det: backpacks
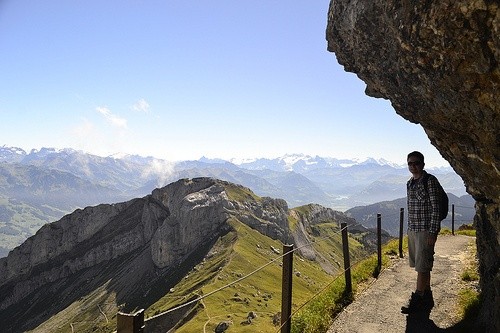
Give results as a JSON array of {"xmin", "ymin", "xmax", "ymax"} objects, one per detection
[{"xmin": 424, "ymin": 174, "xmax": 448, "ymax": 221}]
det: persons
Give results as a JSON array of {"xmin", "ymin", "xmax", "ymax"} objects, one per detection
[{"xmin": 401, "ymin": 151, "xmax": 441, "ymax": 312}]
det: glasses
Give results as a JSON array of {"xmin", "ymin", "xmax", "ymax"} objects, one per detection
[{"xmin": 408, "ymin": 162, "xmax": 420, "ymax": 166}]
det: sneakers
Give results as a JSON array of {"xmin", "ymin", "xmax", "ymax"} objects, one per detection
[{"xmin": 401, "ymin": 291, "xmax": 434, "ymax": 312}]
